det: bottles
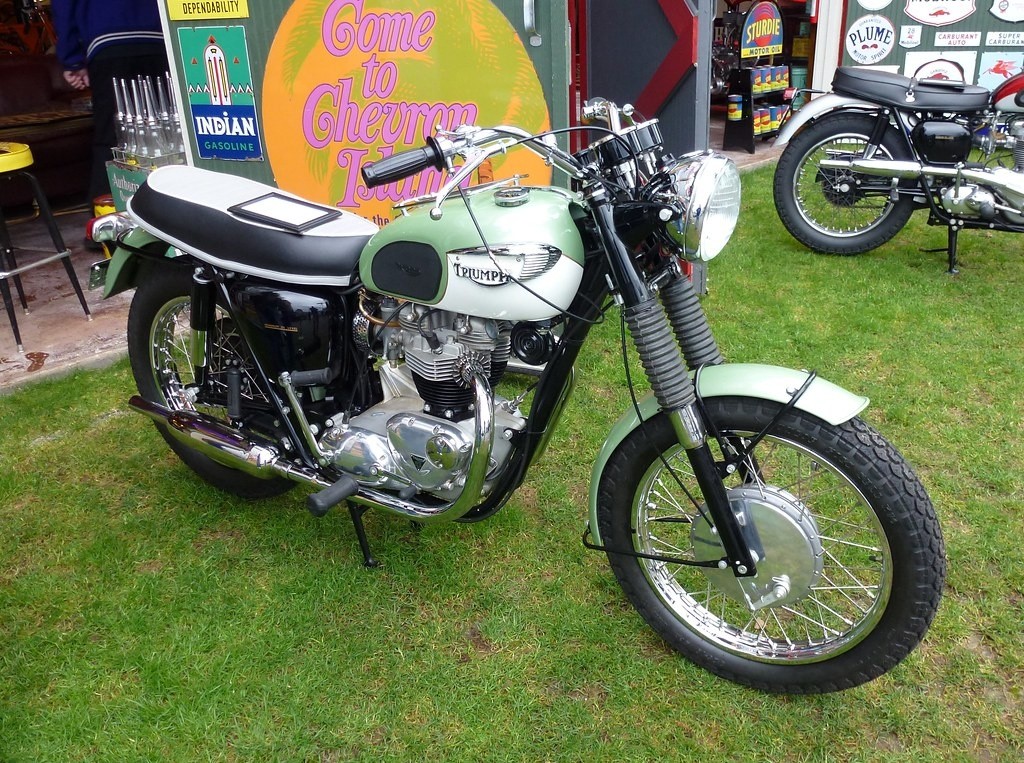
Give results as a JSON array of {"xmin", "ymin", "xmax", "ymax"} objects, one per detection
[{"xmin": 112, "ymin": 72, "xmax": 188, "ymax": 171}]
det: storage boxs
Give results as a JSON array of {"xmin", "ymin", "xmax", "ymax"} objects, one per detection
[
  {"xmin": 104, "ymin": 160, "xmax": 148, "ymax": 213},
  {"xmin": 793, "ymin": 35, "xmax": 811, "ymax": 56}
]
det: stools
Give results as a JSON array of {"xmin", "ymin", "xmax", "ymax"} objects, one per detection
[{"xmin": 0, "ymin": 141, "xmax": 94, "ymax": 353}]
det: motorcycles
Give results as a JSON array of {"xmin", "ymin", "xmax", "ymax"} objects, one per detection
[
  {"xmin": 770, "ymin": 65, "xmax": 1024, "ymax": 274},
  {"xmin": 84, "ymin": 95, "xmax": 947, "ymax": 695}
]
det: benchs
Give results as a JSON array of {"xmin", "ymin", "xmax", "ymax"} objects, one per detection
[{"xmin": 0, "ymin": 53, "xmax": 98, "ymax": 210}]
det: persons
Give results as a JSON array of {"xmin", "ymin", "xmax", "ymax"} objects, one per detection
[{"xmin": 49, "ymin": 0, "xmax": 174, "ymax": 252}]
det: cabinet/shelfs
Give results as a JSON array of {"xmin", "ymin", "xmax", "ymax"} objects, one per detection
[{"xmin": 724, "ymin": 69, "xmax": 793, "ymax": 155}]
